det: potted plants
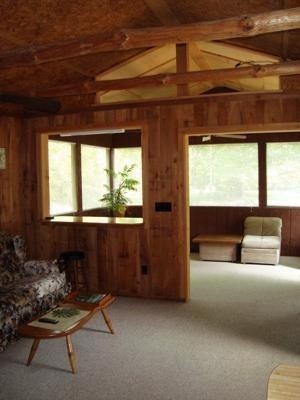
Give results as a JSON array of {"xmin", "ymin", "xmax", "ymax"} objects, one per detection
[{"xmin": 98, "ymin": 164, "xmax": 140, "ymax": 217}]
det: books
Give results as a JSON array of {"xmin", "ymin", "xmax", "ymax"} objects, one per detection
[{"xmin": 76, "ymin": 292, "xmax": 101, "ymax": 304}]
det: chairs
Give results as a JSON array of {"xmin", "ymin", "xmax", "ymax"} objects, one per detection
[{"xmin": 240, "ymin": 215, "xmax": 283, "ymax": 265}]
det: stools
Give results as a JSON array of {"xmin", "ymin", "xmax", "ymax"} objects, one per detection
[{"xmin": 60, "ymin": 251, "xmax": 89, "ymax": 292}]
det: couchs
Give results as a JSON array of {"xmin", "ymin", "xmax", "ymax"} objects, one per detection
[{"xmin": 0, "ymin": 231, "xmax": 72, "ymax": 355}]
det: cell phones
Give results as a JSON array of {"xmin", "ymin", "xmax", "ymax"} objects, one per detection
[{"xmin": 38, "ymin": 318, "xmax": 58, "ymax": 324}]
